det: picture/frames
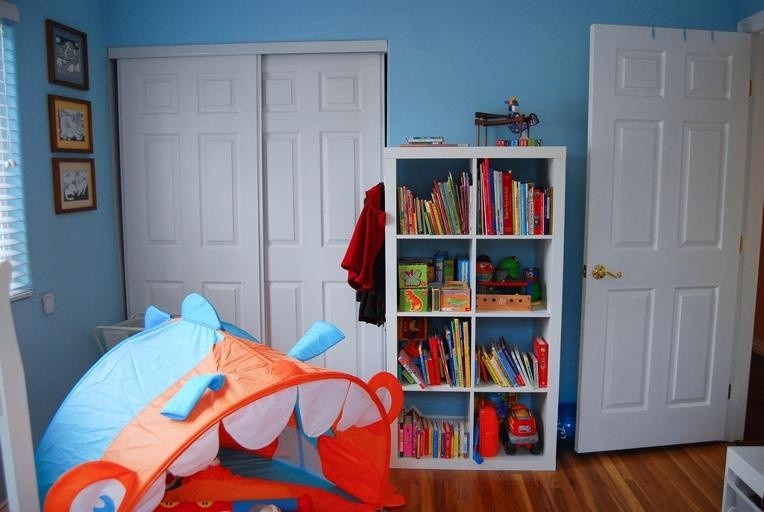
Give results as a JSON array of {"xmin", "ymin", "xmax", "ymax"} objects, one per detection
[{"xmin": 48, "ymin": 20, "xmax": 98, "ymax": 216}]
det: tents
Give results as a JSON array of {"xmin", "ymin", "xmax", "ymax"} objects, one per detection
[{"xmin": 29, "ymin": 289, "xmax": 412, "ymax": 512}]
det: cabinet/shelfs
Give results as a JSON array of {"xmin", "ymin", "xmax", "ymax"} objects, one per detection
[
  {"xmin": 385, "ymin": 146, "xmax": 560, "ymax": 474},
  {"xmin": 112, "ymin": 49, "xmax": 385, "ymax": 405}
]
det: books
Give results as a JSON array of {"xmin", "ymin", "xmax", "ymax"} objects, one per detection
[
  {"xmin": 397, "ymin": 402, "xmax": 468, "ymax": 461},
  {"xmin": 395, "ymin": 136, "xmax": 551, "ymax": 391}
]
